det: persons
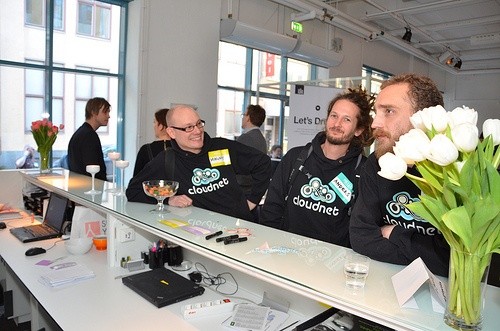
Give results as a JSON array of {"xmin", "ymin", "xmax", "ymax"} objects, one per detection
[
  {"xmin": 125, "ymin": 101, "xmax": 272, "ymax": 224},
  {"xmin": 348, "ymin": 73, "xmax": 500, "ymax": 287},
  {"xmin": 261, "ymin": 84, "xmax": 376, "ymax": 249},
  {"xmin": 132, "ymin": 108, "xmax": 172, "ymax": 178},
  {"xmin": 268, "ymin": 144, "xmax": 282, "ymax": 158},
  {"xmin": 67, "ymin": 97, "xmax": 112, "ymax": 182},
  {"xmin": 236, "ymin": 105, "xmax": 268, "ymax": 156}
]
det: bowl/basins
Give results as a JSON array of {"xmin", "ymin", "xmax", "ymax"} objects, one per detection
[
  {"xmin": 92, "ymin": 235, "xmax": 107, "ymax": 250},
  {"xmin": 66, "ymin": 238, "xmax": 93, "ymax": 255}
]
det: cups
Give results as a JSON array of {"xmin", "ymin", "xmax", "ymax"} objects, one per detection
[{"xmin": 342, "ymin": 252, "xmax": 370, "ymax": 289}]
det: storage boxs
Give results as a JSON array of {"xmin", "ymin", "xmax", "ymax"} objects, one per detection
[{"xmin": 42, "ymin": 198, "xmax": 49, "ymax": 220}]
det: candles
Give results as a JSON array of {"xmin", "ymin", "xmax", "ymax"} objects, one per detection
[
  {"xmin": 108, "ymin": 151, "xmax": 121, "ymax": 160},
  {"xmin": 86, "ymin": 165, "xmax": 100, "ymax": 174},
  {"xmin": 116, "ymin": 159, "xmax": 129, "ymax": 169}
]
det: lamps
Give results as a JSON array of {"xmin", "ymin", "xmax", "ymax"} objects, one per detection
[
  {"xmin": 453, "ymin": 57, "xmax": 463, "ymax": 70},
  {"xmin": 402, "ymin": 26, "xmax": 413, "ymax": 43}
]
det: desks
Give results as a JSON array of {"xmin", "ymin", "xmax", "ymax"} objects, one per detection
[{"xmin": 0, "ymin": 216, "xmax": 312, "ymax": 331}]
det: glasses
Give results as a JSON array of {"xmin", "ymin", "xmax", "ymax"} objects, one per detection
[
  {"xmin": 169, "ymin": 119, "xmax": 206, "ymax": 133},
  {"xmin": 241, "ymin": 114, "xmax": 247, "ymax": 117}
]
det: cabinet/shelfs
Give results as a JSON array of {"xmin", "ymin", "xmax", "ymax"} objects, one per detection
[{"xmin": 23, "ymin": 191, "xmax": 50, "ymax": 217}]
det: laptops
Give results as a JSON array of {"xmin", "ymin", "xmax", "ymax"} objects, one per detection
[
  {"xmin": 121, "ymin": 267, "xmax": 205, "ymax": 308},
  {"xmin": 9, "ymin": 191, "xmax": 69, "ymax": 243}
]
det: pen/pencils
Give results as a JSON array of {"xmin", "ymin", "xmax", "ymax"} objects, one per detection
[{"xmin": 149, "ymin": 240, "xmax": 167, "ymax": 253}]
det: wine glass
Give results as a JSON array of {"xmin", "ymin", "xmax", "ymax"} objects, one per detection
[
  {"xmin": 85, "ymin": 165, "xmax": 102, "ymax": 194},
  {"xmin": 115, "ymin": 160, "xmax": 129, "ymax": 195},
  {"xmin": 142, "ymin": 179, "xmax": 179, "ymax": 214},
  {"xmin": 107, "ymin": 153, "xmax": 121, "ymax": 192}
]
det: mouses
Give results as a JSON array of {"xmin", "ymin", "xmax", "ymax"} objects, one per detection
[
  {"xmin": 25, "ymin": 247, "xmax": 46, "ymax": 257},
  {"xmin": 187, "ymin": 270, "xmax": 202, "ymax": 283}
]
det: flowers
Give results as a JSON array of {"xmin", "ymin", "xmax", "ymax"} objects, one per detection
[
  {"xmin": 374, "ymin": 102, "xmax": 500, "ymax": 325},
  {"xmin": 30, "ymin": 117, "xmax": 65, "ymax": 170}
]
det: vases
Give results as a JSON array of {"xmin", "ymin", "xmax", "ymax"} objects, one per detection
[
  {"xmin": 442, "ymin": 246, "xmax": 492, "ymax": 331},
  {"xmin": 40, "ymin": 149, "xmax": 53, "ymax": 174}
]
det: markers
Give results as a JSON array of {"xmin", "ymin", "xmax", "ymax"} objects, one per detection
[
  {"xmin": 224, "ymin": 237, "xmax": 247, "ymax": 245},
  {"xmin": 215, "ymin": 235, "xmax": 239, "ymax": 242},
  {"xmin": 206, "ymin": 231, "xmax": 223, "ymax": 240}
]
